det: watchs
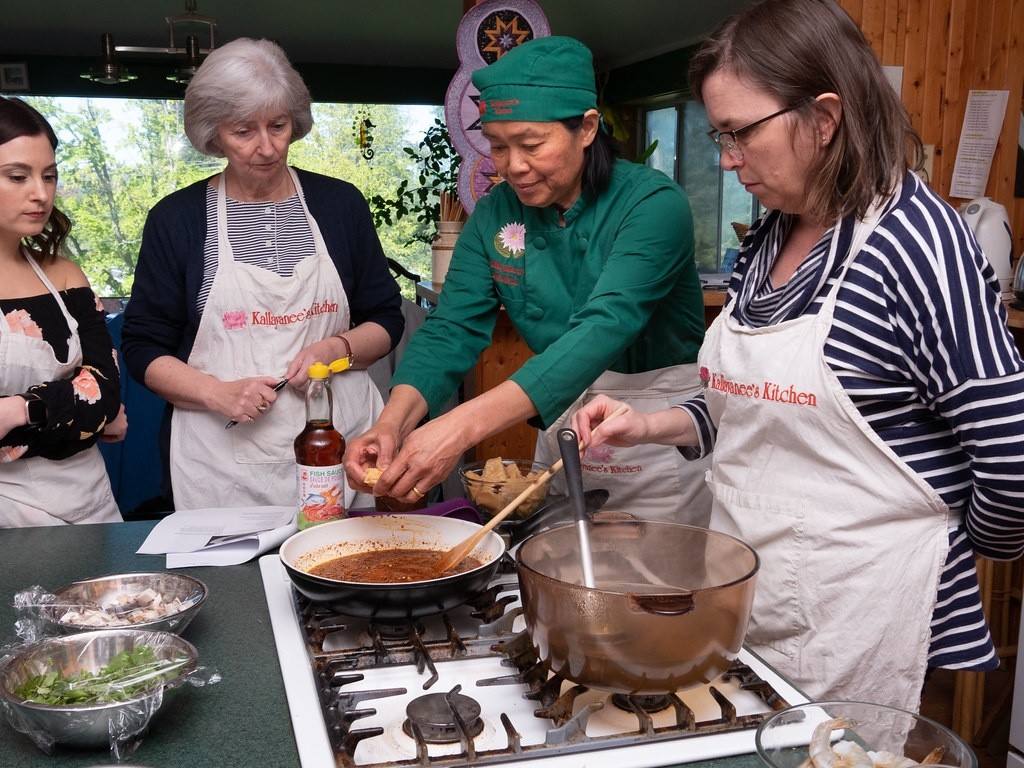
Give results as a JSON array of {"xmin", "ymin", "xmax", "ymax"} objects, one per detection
[
  {"xmin": 336, "ymin": 336, "xmax": 354, "ymax": 368},
  {"xmin": 15, "ymin": 393, "xmax": 47, "ymax": 424}
]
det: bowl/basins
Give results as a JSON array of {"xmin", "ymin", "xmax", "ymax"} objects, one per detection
[
  {"xmin": 42, "ymin": 571, "xmax": 208, "ymax": 637},
  {"xmin": 755, "ymin": 702, "xmax": 978, "ymax": 768},
  {"xmin": 0, "ymin": 629, "xmax": 198, "ymax": 744},
  {"xmin": 459, "ymin": 459, "xmax": 554, "ymax": 523}
]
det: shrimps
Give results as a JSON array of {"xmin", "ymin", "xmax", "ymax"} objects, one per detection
[
  {"xmin": 868, "ymin": 743, "xmax": 960, "ymax": 768},
  {"xmin": 809, "ymin": 715, "xmax": 875, "ymax": 768}
]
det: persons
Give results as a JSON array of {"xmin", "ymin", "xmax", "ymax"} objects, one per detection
[
  {"xmin": 570, "ymin": 0, "xmax": 1024, "ymax": 757},
  {"xmin": 0, "ymin": 96, "xmax": 128, "ymax": 529},
  {"xmin": 345, "ymin": 36, "xmax": 711, "ymax": 528},
  {"xmin": 120, "ymin": 38, "xmax": 406, "ymax": 513}
]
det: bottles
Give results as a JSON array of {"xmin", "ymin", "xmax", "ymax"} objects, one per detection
[
  {"xmin": 432, "ymin": 221, "xmax": 464, "ymax": 286},
  {"xmin": 294, "ymin": 357, "xmax": 352, "ymax": 532}
]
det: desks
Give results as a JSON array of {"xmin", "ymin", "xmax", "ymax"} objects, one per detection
[
  {"xmin": 417, "ymin": 281, "xmax": 725, "ymax": 476},
  {"xmin": 0, "ymin": 511, "xmax": 884, "ymax": 768}
]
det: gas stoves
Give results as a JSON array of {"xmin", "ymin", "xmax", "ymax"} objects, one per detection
[{"xmin": 257, "ymin": 528, "xmax": 841, "ymax": 768}]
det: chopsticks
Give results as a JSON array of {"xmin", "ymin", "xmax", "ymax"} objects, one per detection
[{"xmin": 439, "ymin": 190, "xmax": 463, "ymax": 221}]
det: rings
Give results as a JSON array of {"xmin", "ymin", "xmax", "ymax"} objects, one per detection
[
  {"xmin": 257, "ymin": 400, "xmax": 267, "ymax": 411},
  {"xmin": 247, "ymin": 417, "xmax": 250, "ymax": 424},
  {"xmin": 412, "ymin": 486, "xmax": 425, "ymax": 497}
]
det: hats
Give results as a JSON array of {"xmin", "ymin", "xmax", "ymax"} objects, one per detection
[{"xmin": 472, "ymin": 35, "xmax": 598, "ymax": 123}]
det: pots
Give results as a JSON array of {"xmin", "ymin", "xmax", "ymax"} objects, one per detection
[
  {"xmin": 515, "ymin": 511, "xmax": 760, "ymax": 695},
  {"xmin": 280, "ymin": 489, "xmax": 609, "ymax": 621}
]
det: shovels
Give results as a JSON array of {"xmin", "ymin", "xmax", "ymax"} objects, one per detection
[{"xmin": 434, "ymin": 403, "xmax": 630, "ymax": 572}]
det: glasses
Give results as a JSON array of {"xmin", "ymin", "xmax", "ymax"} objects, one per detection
[{"xmin": 706, "ymin": 100, "xmax": 810, "ymax": 161}]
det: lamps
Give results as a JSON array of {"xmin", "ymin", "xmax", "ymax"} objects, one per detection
[{"xmin": 80, "ymin": 1, "xmax": 225, "ymax": 86}]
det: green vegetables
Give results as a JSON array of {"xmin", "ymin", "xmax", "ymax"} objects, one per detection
[{"xmin": 13, "ymin": 642, "xmax": 180, "ymax": 708}]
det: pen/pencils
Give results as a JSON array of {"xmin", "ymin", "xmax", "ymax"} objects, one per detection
[
  {"xmin": 703, "ymin": 285, "xmax": 728, "ymax": 290},
  {"xmin": 225, "ymin": 379, "xmax": 288, "ymax": 431}
]
